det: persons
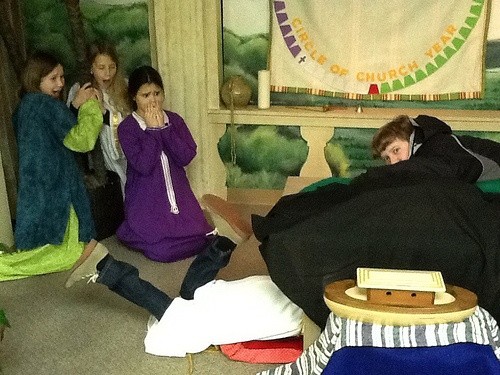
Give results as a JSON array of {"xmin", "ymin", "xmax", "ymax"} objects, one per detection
[
  {"xmin": 66, "ymin": 39, "xmax": 499, "ymax": 357},
  {"xmin": 0, "ymin": 56, "xmax": 104, "ymax": 282}
]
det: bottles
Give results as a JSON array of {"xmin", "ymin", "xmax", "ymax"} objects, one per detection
[{"xmin": 222, "ymin": 75, "xmax": 252, "ymax": 108}]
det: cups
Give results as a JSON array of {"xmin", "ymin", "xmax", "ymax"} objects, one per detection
[{"xmin": 258, "ymin": 69, "xmax": 271, "ymax": 109}]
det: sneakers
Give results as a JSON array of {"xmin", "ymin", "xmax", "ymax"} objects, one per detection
[
  {"xmin": 63, "ymin": 238, "xmax": 109, "ymax": 289},
  {"xmin": 201, "ymin": 193, "xmax": 254, "ymax": 248}
]
